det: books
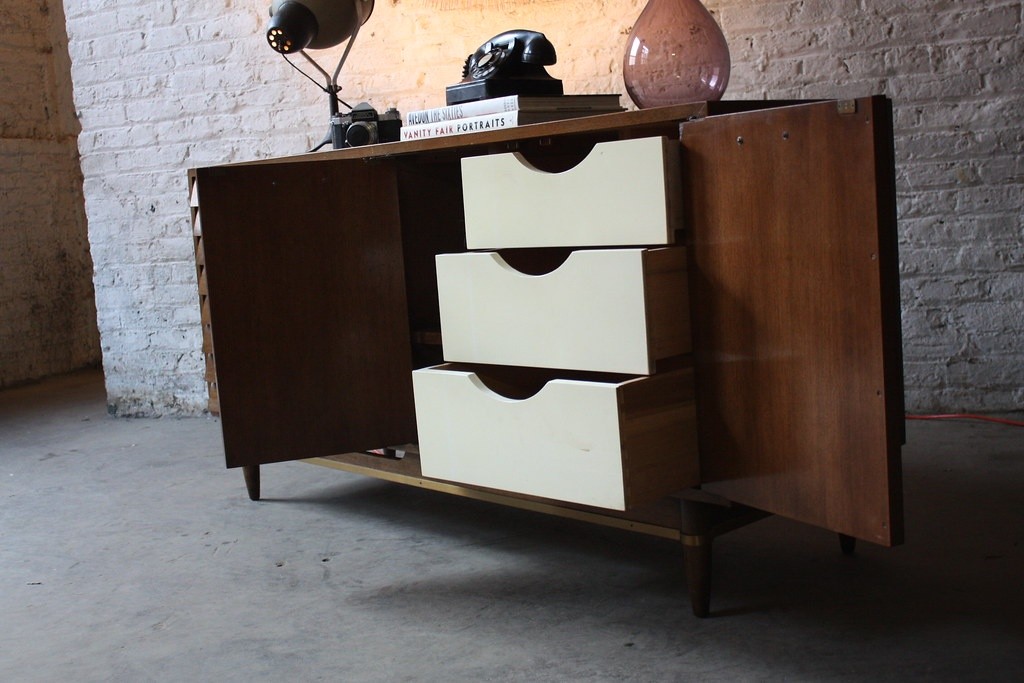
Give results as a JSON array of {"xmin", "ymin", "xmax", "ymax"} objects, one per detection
[{"xmin": 400, "ymin": 94, "xmax": 628, "ymax": 142}]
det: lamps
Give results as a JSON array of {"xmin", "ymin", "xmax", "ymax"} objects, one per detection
[{"xmin": 265, "ymin": 0, "xmax": 375, "ymax": 152}]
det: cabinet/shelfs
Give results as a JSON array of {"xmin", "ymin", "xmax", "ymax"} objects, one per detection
[{"xmin": 185, "ymin": 95, "xmax": 909, "ymax": 620}]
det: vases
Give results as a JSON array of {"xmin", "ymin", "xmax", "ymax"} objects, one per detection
[{"xmin": 622, "ymin": 0, "xmax": 731, "ymax": 109}]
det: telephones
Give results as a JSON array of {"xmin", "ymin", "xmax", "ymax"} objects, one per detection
[{"xmin": 446, "ymin": 30, "xmax": 564, "ymax": 105}]
